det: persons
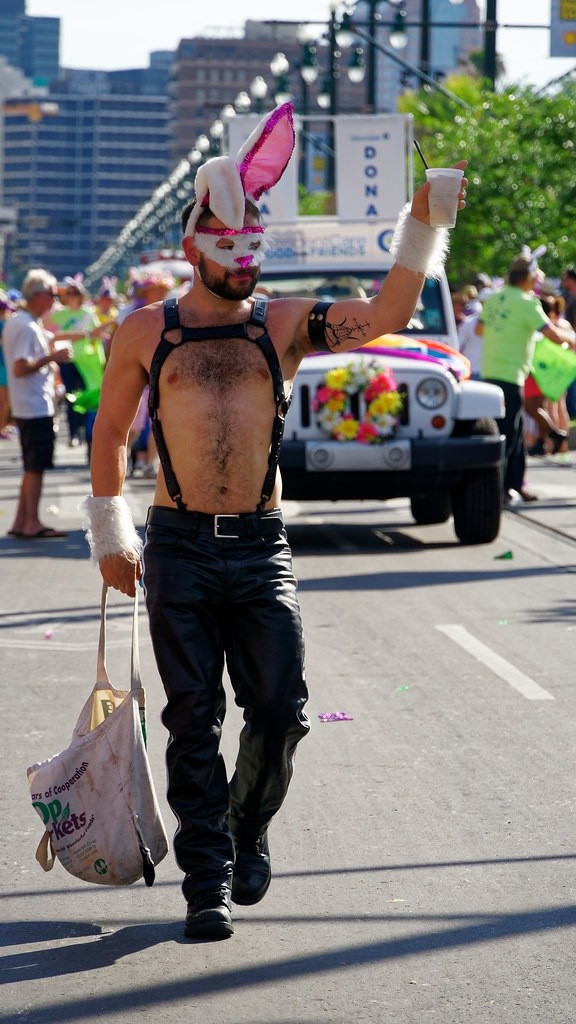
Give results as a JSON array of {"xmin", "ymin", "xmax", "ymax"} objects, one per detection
[
  {"xmin": 85, "ymin": 159, "xmax": 470, "ymax": 939},
  {"xmin": 0, "ymin": 253, "xmax": 576, "ymax": 539}
]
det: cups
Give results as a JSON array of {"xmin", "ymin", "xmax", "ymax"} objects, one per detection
[
  {"xmin": 426, "ymin": 167, "xmax": 464, "ymax": 228},
  {"xmin": 54, "ymin": 339, "xmax": 70, "ymax": 351}
]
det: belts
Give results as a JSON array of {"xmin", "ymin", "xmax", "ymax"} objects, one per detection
[{"xmin": 146, "ymin": 506, "xmax": 283, "ymax": 538}]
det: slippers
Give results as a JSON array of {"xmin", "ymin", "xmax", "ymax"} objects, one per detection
[{"xmin": 7, "ymin": 527, "xmax": 67, "ymax": 538}]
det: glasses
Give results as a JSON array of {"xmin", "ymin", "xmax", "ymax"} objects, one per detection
[{"xmin": 50, "ymin": 291, "xmax": 61, "ymax": 300}]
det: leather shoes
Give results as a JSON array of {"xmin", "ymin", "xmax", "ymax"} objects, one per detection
[
  {"xmin": 183, "ymin": 874, "xmax": 234, "ymax": 937},
  {"xmin": 227, "ymin": 824, "xmax": 271, "ymax": 904}
]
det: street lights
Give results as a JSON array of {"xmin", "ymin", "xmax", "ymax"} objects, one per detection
[{"xmin": 84, "ymin": 0, "xmax": 409, "ymax": 281}]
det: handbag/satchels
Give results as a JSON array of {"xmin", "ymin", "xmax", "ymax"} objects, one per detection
[{"xmin": 26, "ymin": 581, "xmax": 170, "ymax": 888}]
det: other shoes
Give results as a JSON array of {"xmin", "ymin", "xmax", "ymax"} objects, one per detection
[
  {"xmin": 505, "ymin": 486, "xmax": 539, "ymax": 503},
  {"xmin": 130, "ymin": 461, "xmax": 157, "ymax": 480}
]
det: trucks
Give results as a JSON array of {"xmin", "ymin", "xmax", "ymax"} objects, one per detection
[{"xmin": 220, "ymin": 112, "xmax": 509, "ymax": 545}]
